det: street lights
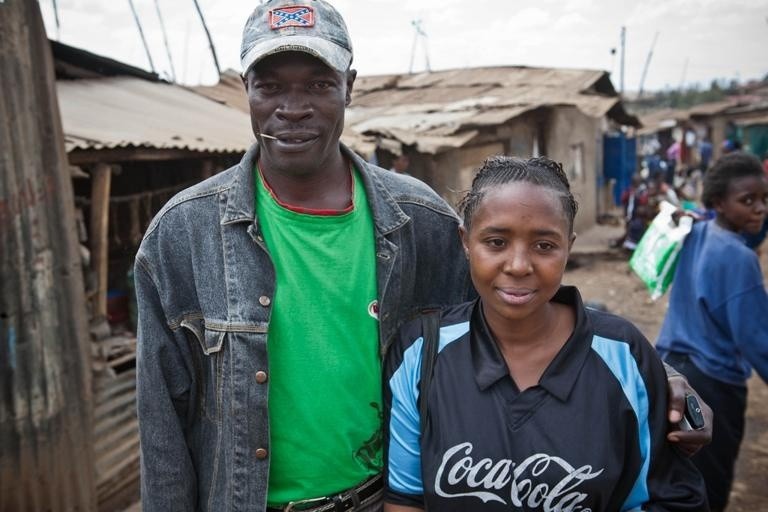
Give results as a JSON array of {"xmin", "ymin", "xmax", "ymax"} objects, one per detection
[{"xmin": 629, "ymin": 203, "xmax": 693, "ymax": 301}]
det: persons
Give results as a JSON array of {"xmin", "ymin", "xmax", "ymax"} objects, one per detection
[
  {"xmin": 131, "ymin": 0, "xmax": 718, "ymax": 512},
  {"xmin": 376, "ymin": 151, "xmax": 719, "ymax": 512},
  {"xmin": 649, "ymin": 153, "xmax": 768, "ymax": 510}
]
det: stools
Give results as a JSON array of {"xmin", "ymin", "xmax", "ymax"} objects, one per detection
[{"xmin": 267, "ymin": 472, "xmax": 383, "ymax": 512}]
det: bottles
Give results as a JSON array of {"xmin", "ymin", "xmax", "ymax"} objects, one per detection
[{"xmin": 240, "ymin": 0, "xmax": 352, "ymax": 78}]
